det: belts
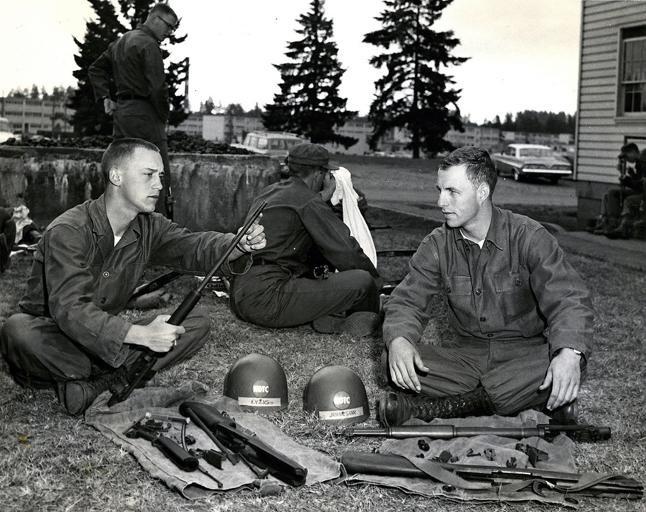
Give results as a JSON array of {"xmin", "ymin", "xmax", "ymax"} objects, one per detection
[{"xmin": 232, "ymin": 258, "xmax": 278, "ymax": 271}]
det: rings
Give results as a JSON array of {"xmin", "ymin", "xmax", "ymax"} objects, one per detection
[{"xmin": 174, "ymin": 340, "xmax": 177, "ymax": 346}]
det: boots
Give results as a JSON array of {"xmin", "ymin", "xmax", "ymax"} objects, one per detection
[
  {"xmin": 508, "ymin": 398, "xmax": 579, "ymax": 425},
  {"xmin": 54, "ymin": 356, "xmax": 152, "ymax": 416},
  {"xmin": 585, "ymin": 215, "xmax": 632, "ymax": 240},
  {"xmin": 378, "ymin": 384, "xmax": 496, "ymax": 428}
]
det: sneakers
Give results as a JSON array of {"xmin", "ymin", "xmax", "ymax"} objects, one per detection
[{"xmin": 313, "ymin": 311, "xmax": 380, "ymax": 336}]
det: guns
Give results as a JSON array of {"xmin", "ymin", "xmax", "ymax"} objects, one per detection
[
  {"xmin": 341, "ymin": 417, "xmax": 610, "ymax": 443},
  {"xmin": 165, "ymin": 186, "xmax": 174, "ymax": 222},
  {"xmin": 107, "ymin": 201, "xmax": 267, "ymax": 409},
  {"xmin": 618, "ymin": 155, "xmax": 627, "ymax": 212},
  {"xmin": 341, "ymin": 452, "xmax": 644, "ymax": 499}
]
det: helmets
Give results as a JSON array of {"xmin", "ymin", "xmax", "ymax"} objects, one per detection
[
  {"xmin": 306, "ymin": 365, "xmax": 368, "ymax": 424},
  {"xmin": 223, "ymin": 352, "xmax": 289, "ymax": 412}
]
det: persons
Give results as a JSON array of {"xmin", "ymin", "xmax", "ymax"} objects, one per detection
[
  {"xmin": 88, "ymin": 4, "xmax": 177, "ymax": 219},
  {"xmin": 379, "ymin": 146, "xmax": 594, "ymax": 427},
  {"xmin": 232, "ymin": 143, "xmax": 383, "ymax": 337},
  {"xmin": 0, "ymin": 138, "xmax": 266, "ymax": 415},
  {"xmin": 585, "ymin": 143, "xmax": 646, "ymax": 240}
]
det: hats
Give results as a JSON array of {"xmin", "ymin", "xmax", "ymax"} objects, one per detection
[{"xmin": 286, "ymin": 144, "xmax": 339, "ymax": 171}]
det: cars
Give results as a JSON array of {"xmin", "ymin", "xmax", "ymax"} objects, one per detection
[
  {"xmin": 228, "ymin": 129, "xmax": 303, "ymax": 155},
  {"xmin": 488, "ymin": 144, "xmax": 572, "ymax": 182}
]
